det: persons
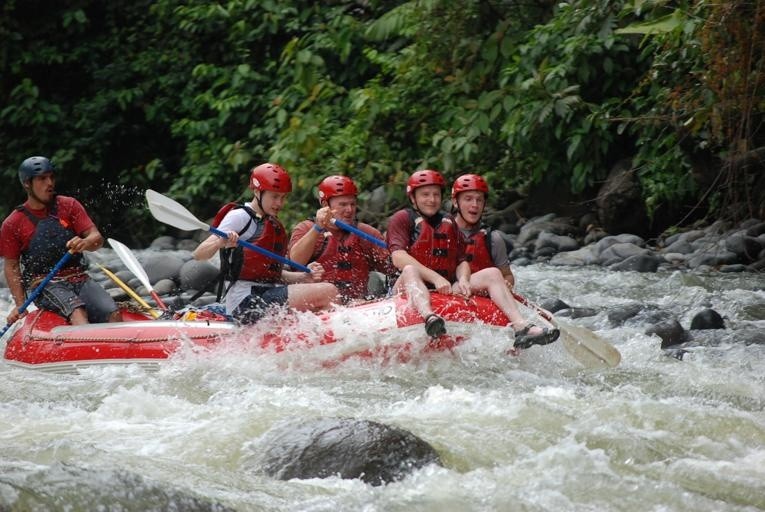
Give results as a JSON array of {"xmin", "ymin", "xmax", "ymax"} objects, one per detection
[
  {"xmin": 192, "ymin": 162, "xmax": 338, "ymax": 323},
  {"xmin": 288, "ymin": 174, "xmax": 397, "ymax": 303},
  {"xmin": 0, "ymin": 155, "xmax": 123, "ymax": 327},
  {"xmin": 386, "ymin": 170, "xmax": 560, "ymax": 349},
  {"xmin": 450, "ymin": 173, "xmax": 515, "ymax": 290}
]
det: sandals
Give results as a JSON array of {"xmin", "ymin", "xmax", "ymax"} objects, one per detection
[
  {"xmin": 425, "ymin": 314, "xmax": 447, "ymax": 339},
  {"xmin": 514, "ymin": 323, "xmax": 560, "ymax": 349}
]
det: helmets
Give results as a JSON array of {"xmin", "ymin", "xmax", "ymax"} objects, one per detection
[
  {"xmin": 319, "ymin": 175, "xmax": 357, "ymax": 201},
  {"xmin": 407, "ymin": 170, "xmax": 445, "ymax": 195},
  {"xmin": 249, "ymin": 163, "xmax": 292, "ymax": 193},
  {"xmin": 452, "ymin": 174, "xmax": 489, "ymax": 197},
  {"xmin": 19, "ymin": 156, "xmax": 54, "ymax": 184}
]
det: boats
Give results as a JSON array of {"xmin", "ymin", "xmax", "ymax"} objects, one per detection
[{"xmin": 4, "ymin": 286, "xmax": 520, "ymax": 371}]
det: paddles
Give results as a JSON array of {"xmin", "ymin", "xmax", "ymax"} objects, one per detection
[
  {"xmin": 510, "ymin": 291, "xmax": 621, "ymax": 368},
  {"xmin": 146, "ymin": 189, "xmax": 312, "ymax": 274},
  {"xmin": 107, "ymin": 238, "xmax": 167, "ymax": 312}
]
